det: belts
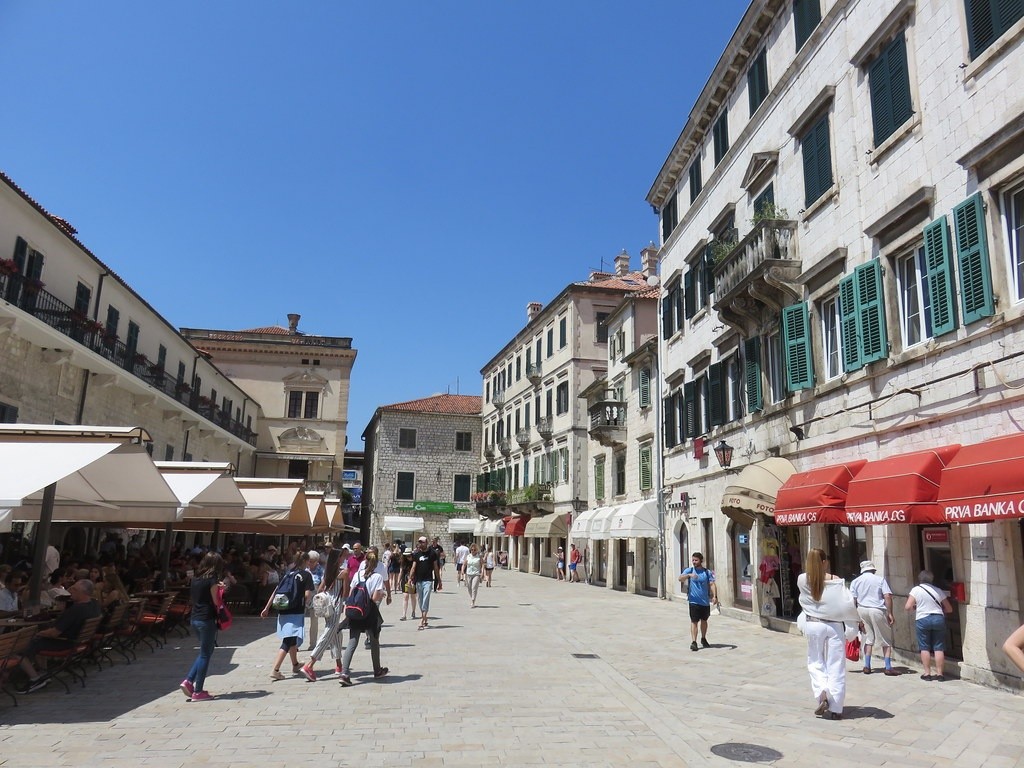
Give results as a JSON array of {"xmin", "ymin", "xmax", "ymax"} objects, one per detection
[{"xmin": 930, "ymin": 614, "xmax": 942, "ymax": 615}]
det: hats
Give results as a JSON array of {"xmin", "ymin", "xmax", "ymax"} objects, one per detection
[
  {"xmin": 860, "ymin": 561, "xmax": 877, "ymax": 573},
  {"xmin": 558, "ymin": 546, "xmax": 563, "ymax": 550},
  {"xmin": 324, "ymin": 542, "xmax": 350, "ymax": 549},
  {"xmin": 403, "ymin": 537, "xmax": 427, "ymax": 555}
]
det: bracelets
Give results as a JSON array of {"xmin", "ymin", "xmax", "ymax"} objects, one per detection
[
  {"xmin": 862, "ymin": 623, "xmax": 864, "ymax": 627},
  {"xmin": 713, "ymin": 596, "xmax": 717, "ymax": 598}
]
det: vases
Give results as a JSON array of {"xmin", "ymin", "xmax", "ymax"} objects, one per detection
[{"xmin": 475, "ymin": 501, "xmax": 506, "ymax": 507}]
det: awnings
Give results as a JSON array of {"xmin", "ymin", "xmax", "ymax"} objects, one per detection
[
  {"xmin": 448, "ymin": 512, "xmax": 568, "ymax": 538},
  {"xmin": 382, "ymin": 516, "xmax": 424, "ymax": 532},
  {"xmin": 720, "ymin": 432, "xmax": 1024, "ymax": 527},
  {"xmin": 570, "ymin": 498, "xmax": 658, "ymax": 540}
]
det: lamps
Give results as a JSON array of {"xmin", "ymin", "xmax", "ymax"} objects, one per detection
[
  {"xmin": 436, "ymin": 468, "xmax": 441, "ymax": 484},
  {"xmin": 571, "ymin": 497, "xmax": 583, "ymax": 513},
  {"xmin": 713, "ymin": 441, "xmax": 743, "ymax": 476}
]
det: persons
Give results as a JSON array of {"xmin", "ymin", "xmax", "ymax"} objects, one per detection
[
  {"xmin": 678, "ymin": 552, "xmax": 718, "ymax": 651},
  {"xmin": 569, "ymin": 543, "xmax": 581, "ymax": 583},
  {"xmin": 498, "ymin": 552, "xmax": 507, "ymax": 564},
  {"xmin": 0, "ymin": 531, "xmax": 445, "ymax": 695},
  {"xmin": 556, "ymin": 546, "xmax": 565, "ymax": 581},
  {"xmin": 796, "ymin": 548, "xmax": 868, "ymax": 721},
  {"xmin": 455, "ymin": 540, "xmax": 495, "ymax": 608},
  {"xmin": 1001, "ymin": 624, "xmax": 1024, "ymax": 672},
  {"xmin": 180, "ymin": 550, "xmax": 228, "ymax": 702},
  {"xmin": 849, "ymin": 560, "xmax": 903, "ymax": 675},
  {"xmin": 905, "ymin": 570, "xmax": 953, "ymax": 682}
]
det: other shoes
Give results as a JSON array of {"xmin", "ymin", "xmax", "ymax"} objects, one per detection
[
  {"xmin": 921, "ymin": 674, "xmax": 944, "ymax": 681},
  {"xmin": 690, "ymin": 638, "xmax": 710, "ymax": 650},
  {"xmin": 884, "ymin": 669, "xmax": 902, "ymax": 675},
  {"xmin": 400, "ymin": 611, "xmax": 415, "ymax": 620},
  {"xmin": 417, "ymin": 622, "xmax": 428, "ymax": 630},
  {"xmin": 863, "ymin": 667, "xmax": 871, "ymax": 674},
  {"xmin": 815, "ymin": 699, "xmax": 841, "ymax": 719}
]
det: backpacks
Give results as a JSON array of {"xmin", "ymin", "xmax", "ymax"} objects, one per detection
[
  {"xmin": 272, "ymin": 568, "xmax": 302, "ymax": 614},
  {"xmin": 214, "ymin": 581, "xmax": 232, "ymax": 631},
  {"xmin": 345, "ymin": 571, "xmax": 378, "ymax": 629},
  {"xmin": 313, "ymin": 570, "xmax": 345, "ymax": 618},
  {"xmin": 575, "ymin": 549, "xmax": 582, "ymax": 563}
]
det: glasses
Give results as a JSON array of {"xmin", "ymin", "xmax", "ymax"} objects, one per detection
[{"xmin": 470, "ymin": 548, "xmax": 476, "ymax": 552}]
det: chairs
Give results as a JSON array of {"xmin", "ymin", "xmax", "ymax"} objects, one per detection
[{"xmin": 0, "ymin": 581, "xmax": 280, "ymax": 707}]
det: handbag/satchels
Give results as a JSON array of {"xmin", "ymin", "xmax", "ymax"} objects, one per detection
[
  {"xmin": 846, "ymin": 636, "xmax": 861, "ymax": 662},
  {"xmin": 405, "ymin": 583, "xmax": 415, "ymax": 593},
  {"xmin": 558, "ymin": 562, "xmax": 563, "ymax": 569}
]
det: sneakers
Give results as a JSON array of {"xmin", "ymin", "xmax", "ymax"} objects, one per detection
[
  {"xmin": 293, "ymin": 660, "xmax": 306, "ymax": 673},
  {"xmin": 335, "ymin": 666, "xmax": 351, "ymax": 674},
  {"xmin": 180, "ymin": 679, "xmax": 213, "ymax": 701},
  {"xmin": 373, "ymin": 667, "xmax": 388, "ymax": 680},
  {"xmin": 300, "ymin": 665, "xmax": 316, "ymax": 682},
  {"xmin": 339, "ymin": 671, "xmax": 353, "ymax": 686},
  {"xmin": 270, "ymin": 670, "xmax": 285, "ymax": 680},
  {"xmin": 18, "ymin": 672, "xmax": 52, "ymax": 694}
]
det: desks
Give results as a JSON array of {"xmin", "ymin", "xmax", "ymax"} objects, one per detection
[
  {"xmin": 136, "ymin": 589, "xmax": 179, "ymax": 595},
  {"xmin": 0, "ymin": 618, "xmax": 57, "ymax": 626},
  {"xmin": 166, "ymin": 585, "xmax": 192, "ymax": 589},
  {"xmin": 128, "ymin": 597, "xmax": 148, "ymax": 604}
]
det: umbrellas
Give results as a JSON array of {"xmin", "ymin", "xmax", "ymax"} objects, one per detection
[{"xmin": 0, "ymin": 422, "xmax": 361, "ymax": 606}]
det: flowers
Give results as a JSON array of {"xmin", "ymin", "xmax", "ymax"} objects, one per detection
[
  {"xmin": 24, "ymin": 277, "xmax": 46, "ymax": 294},
  {"xmin": 68, "ymin": 309, "xmax": 121, "ymax": 344},
  {"xmin": 134, "ymin": 352, "xmax": 229, "ymax": 420},
  {"xmin": 470, "ymin": 491, "xmax": 506, "ymax": 502},
  {"xmin": 0, "ymin": 257, "xmax": 17, "ymax": 277}
]
